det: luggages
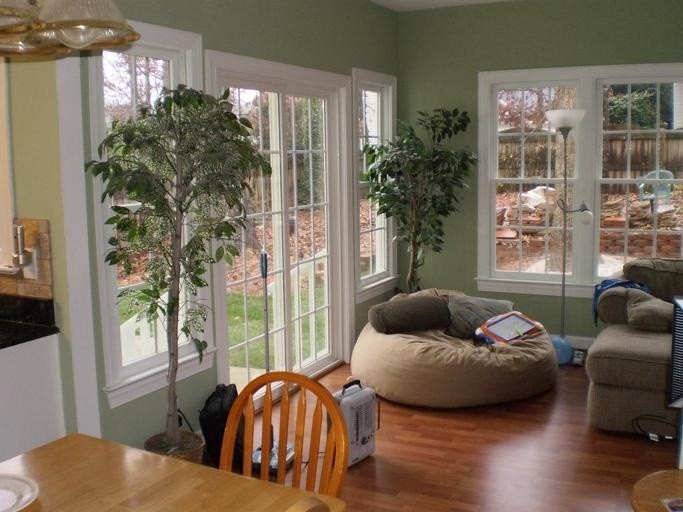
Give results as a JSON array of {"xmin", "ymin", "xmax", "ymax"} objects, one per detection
[
  {"xmin": 327, "ymin": 376, "xmax": 380, "ymax": 469},
  {"xmin": 199, "ymin": 383, "xmax": 244, "ymax": 469}
]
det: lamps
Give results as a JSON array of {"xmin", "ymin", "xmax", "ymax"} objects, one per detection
[
  {"xmin": 541, "ymin": 108, "xmax": 594, "ymax": 337},
  {"xmin": 0, "ymin": 0, "xmax": 139, "ymax": 58}
]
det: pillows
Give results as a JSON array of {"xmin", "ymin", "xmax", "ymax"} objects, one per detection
[
  {"xmin": 367, "ymin": 293, "xmax": 514, "ymax": 339},
  {"xmin": 624, "ymin": 285, "xmax": 675, "ymax": 331}
]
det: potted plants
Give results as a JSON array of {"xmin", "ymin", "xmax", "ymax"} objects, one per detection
[{"xmin": 82, "ymin": 85, "xmax": 275, "ymax": 464}]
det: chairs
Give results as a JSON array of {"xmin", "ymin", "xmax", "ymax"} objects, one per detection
[
  {"xmin": 637, "ymin": 170, "xmax": 675, "ymax": 213},
  {"xmin": 217, "ymin": 371, "xmax": 349, "ymax": 497}
]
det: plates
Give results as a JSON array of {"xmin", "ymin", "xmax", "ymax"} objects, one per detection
[{"xmin": 0, "ymin": 472, "xmax": 40, "ymax": 512}]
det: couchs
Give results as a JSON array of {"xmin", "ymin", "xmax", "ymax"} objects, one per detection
[{"xmin": 581, "ymin": 258, "xmax": 681, "ymax": 438}]
